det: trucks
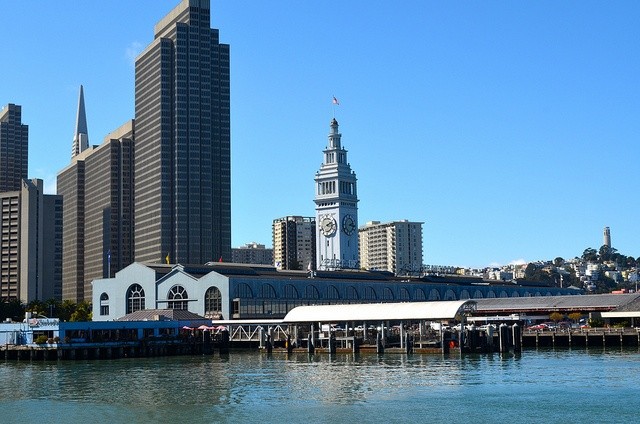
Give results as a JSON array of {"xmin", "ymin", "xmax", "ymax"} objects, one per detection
[
  {"xmin": 430, "ymin": 323, "xmax": 450, "ymax": 331},
  {"xmin": 322, "ymin": 324, "xmax": 342, "ymax": 332}
]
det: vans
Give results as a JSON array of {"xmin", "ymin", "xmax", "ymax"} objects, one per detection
[{"xmin": 479, "ymin": 325, "xmax": 493, "ymax": 331}]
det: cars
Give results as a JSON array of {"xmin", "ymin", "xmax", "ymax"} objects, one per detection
[{"xmin": 452, "ymin": 323, "xmax": 468, "ymax": 331}]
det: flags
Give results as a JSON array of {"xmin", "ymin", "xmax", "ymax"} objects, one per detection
[
  {"xmin": 308, "ymin": 262, "xmax": 311, "ymax": 269},
  {"xmin": 106, "ymin": 249, "xmax": 111, "ymax": 262},
  {"xmin": 166, "ymin": 255, "xmax": 169, "ymax": 264},
  {"xmin": 277, "ymin": 262, "xmax": 279, "ymax": 266},
  {"xmin": 219, "ymin": 257, "xmax": 222, "ymax": 261},
  {"xmin": 333, "ymin": 97, "xmax": 339, "ymax": 104}
]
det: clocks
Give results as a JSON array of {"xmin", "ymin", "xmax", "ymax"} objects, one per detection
[
  {"xmin": 342, "ymin": 213, "xmax": 356, "ymax": 235},
  {"xmin": 319, "ymin": 214, "xmax": 336, "ymax": 237}
]
